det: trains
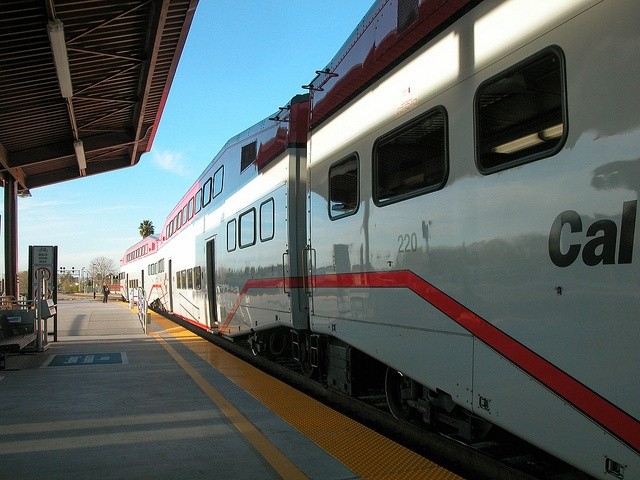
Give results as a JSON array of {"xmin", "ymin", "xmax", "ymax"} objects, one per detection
[{"xmin": 118, "ymin": 0, "xmax": 639, "ymax": 478}]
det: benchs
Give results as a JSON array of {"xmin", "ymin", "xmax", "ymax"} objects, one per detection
[{"xmin": 0, "ymin": 313, "xmax": 37, "ymax": 372}]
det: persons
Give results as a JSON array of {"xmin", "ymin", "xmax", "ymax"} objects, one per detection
[{"xmin": 102, "ymin": 284, "xmax": 110, "ymax": 303}]
